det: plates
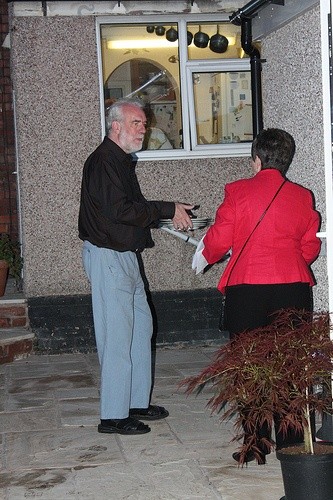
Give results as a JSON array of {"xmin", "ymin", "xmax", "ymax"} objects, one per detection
[{"xmin": 162, "ymin": 215, "xmax": 209, "ymax": 230}]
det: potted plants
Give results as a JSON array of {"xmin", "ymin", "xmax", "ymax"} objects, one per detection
[
  {"xmin": 0, "ymin": 231, "xmax": 25, "ymax": 297},
  {"xmin": 176, "ymin": 307, "xmax": 333, "ymax": 500}
]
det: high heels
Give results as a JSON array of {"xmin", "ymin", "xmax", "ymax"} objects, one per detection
[{"xmin": 233, "ymin": 447, "xmax": 273, "ymax": 464}]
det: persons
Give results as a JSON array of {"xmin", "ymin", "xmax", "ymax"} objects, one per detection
[
  {"xmin": 78, "ymin": 98, "xmax": 195, "ymax": 435},
  {"xmin": 202, "ymin": 128, "xmax": 322, "ymax": 466}
]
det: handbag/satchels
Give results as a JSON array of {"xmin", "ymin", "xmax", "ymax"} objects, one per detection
[{"xmin": 217, "ymin": 290, "xmax": 229, "ymax": 332}]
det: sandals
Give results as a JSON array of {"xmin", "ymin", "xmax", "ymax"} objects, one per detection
[
  {"xmin": 129, "ymin": 404, "xmax": 169, "ymax": 421},
  {"xmin": 97, "ymin": 417, "xmax": 150, "ymax": 435}
]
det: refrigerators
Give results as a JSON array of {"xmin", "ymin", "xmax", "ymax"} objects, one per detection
[{"xmin": 211, "ymin": 71, "xmax": 255, "ymax": 144}]
